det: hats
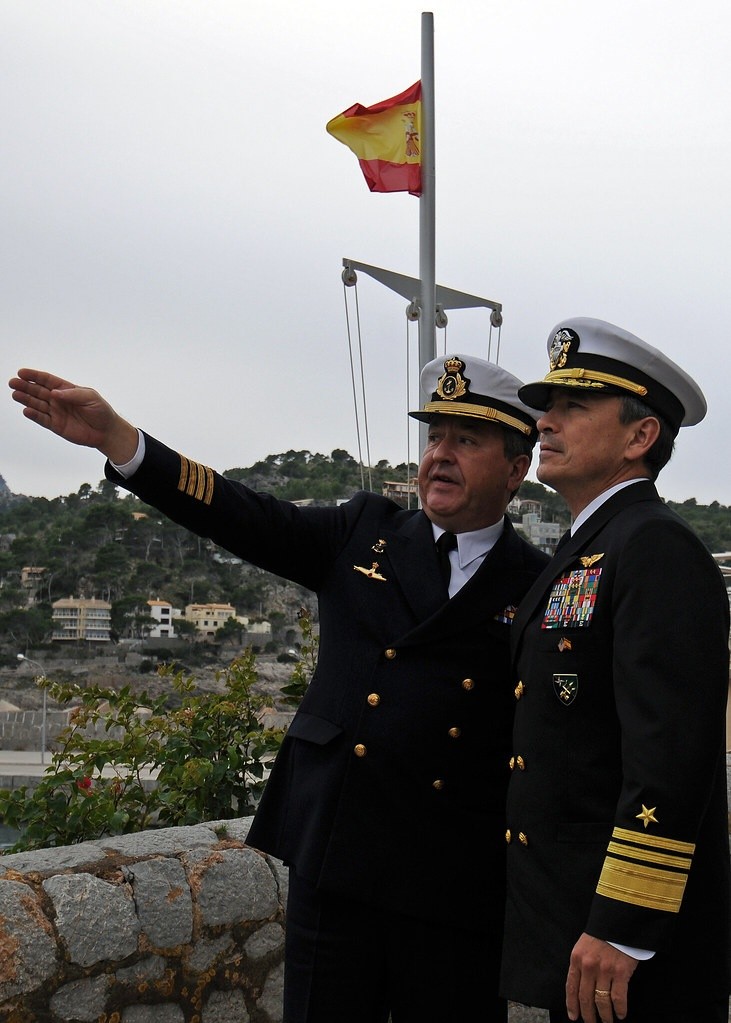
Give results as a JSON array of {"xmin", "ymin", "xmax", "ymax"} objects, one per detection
[
  {"xmin": 407, "ymin": 353, "xmax": 546, "ymax": 442},
  {"xmin": 517, "ymin": 316, "xmax": 707, "ymax": 427}
]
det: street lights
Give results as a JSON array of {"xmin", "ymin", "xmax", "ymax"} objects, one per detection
[{"xmin": 18, "ymin": 654, "xmax": 46, "ymax": 764}]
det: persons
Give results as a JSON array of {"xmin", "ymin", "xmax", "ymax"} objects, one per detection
[
  {"xmin": 6, "ymin": 353, "xmax": 549, "ymax": 1023},
  {"xmin": 512, "ymin": 319, "xmax": 730, "ymax": 1023}
]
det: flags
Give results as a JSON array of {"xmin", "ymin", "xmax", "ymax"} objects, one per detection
[{"xmin": 325, "ymin": 78, "xmax": 423, "ymax": 197}]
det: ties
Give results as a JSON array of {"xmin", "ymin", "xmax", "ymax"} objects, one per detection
[
  {"xmin": 554, "ymin": 529, "xmax": 571, "ymax": 558},
  {"xmin": 435, "ymin": 531, "xmax": 458, "ymax": 601}
]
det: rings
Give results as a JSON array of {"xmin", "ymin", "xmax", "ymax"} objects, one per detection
[{"xmin": 594, "ymin": 990, "xmax": 611, "ymax": 997}]
